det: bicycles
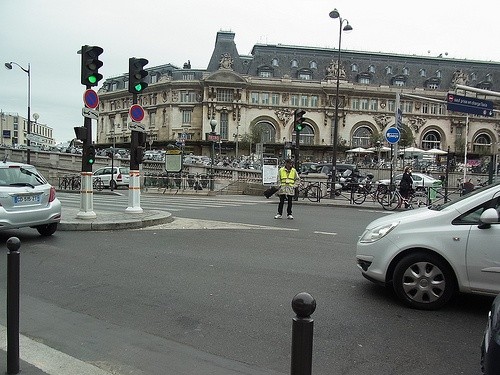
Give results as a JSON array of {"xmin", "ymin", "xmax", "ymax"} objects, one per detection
[
  {"xmin": 351, "ymin": 178, "xmax": 393, "ymax": 206},
  {"xmin": 381, "ymin": 182, "xmax": 431, "ymax": 210},
  {"xmin": 285, "ymin": 182, "xmax": 322, "ymax": 202},
  {"xmin": 60, "ymin": 175, "xmax": 104, "ymax": 191}
]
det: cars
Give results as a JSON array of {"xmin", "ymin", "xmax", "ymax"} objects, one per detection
[
  {"xmin": 479, "ymin": 267, "xmax": 500, "ymax": 374},
  {"xmin": 375, "ymin": 173, "xmax": 442, "ymax": 194},
  {"xmin": 92, "ymin": 167, "xmax": 129, "ymax": 189},
  {"xmin": 70, "ymin": 144, "xmax": 129, "ymax": 157},
  {"xmin": 355, "ymin": 181, "xmax": 500, "ymax": 310},
  {"xmin": 0, "ymin": 161, "xmax": 61, "ymax": 236}
]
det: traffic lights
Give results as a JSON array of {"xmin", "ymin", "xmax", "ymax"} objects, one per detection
[
  {"xmin": 129, "ymin": 58, "xmax": 148, "ymax": 94},
  {"xmin": 138, "ymin": 132, "xmax": 146, "ymax": 147},
  {"xmin": 74, "ymin": 127, "xmax": 87, "ymax": 141},
  {"xmin": 81, "ymin": 45, "xmax": 104, "ymax": 86},
  {"xmin": 294, "ymin": 109, "xmax": 306, "ymax": 131},
  {"xmin": 87, "ymin": 145, "xmax": 95, "ymax": 165}
]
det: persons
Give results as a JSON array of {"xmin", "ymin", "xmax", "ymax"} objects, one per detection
[
  {"xmin": 397, "ymin": 166, "xmax": 413, "ymax": 208},
  {"xmin": 275, "ymin": 159, "xmax": 301, "ymax": 220}
]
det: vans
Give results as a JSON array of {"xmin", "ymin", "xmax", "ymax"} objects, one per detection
[{"xmin": 301, "ymin": 164, "xmax": 359, "ymax": 189}]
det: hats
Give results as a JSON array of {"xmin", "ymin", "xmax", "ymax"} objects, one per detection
[{"xmin": 284, "ymin": 159, "xmax": 294, "ymax": 164}]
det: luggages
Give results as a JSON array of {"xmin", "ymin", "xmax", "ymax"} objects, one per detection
[{"xmin": 263, "ymin": 185, "xmax": 281, "ymax": 199}]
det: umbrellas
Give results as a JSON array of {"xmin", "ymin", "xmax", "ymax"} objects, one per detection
[{"xmin": 346, "ymin": 145, "xmax": 447, "ymax": 164}]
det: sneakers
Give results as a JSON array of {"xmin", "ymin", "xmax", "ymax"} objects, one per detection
[
  {"xmin": 287, "ymin": 215, "xmax": 294, "ymax": 219},
  {"xmin": 274, "ymin": 214, "xmax": 283, "ymax": 219}
]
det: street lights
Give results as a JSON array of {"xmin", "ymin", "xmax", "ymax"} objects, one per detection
[
  {"xmin": 329, "ymin": 9, "xmax": 353, "ymax": 200},
  {"xmin": 5, "ymin": 62, "xmax": 30, "ymax": 165},
  {"xmin": 210, "ymin": 119, "xmax": 218, "ymax": 191}
]
